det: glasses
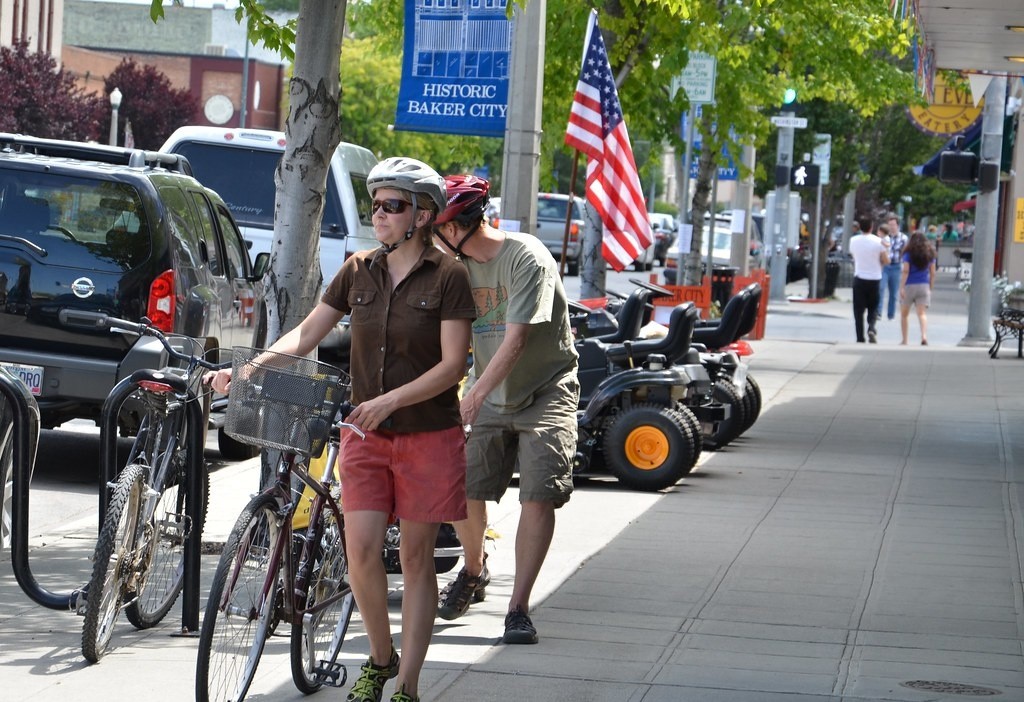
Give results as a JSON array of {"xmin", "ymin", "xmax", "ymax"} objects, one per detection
[{"xmin": 370, "ymin": 199, "xmax": 426, "ymax": 215}]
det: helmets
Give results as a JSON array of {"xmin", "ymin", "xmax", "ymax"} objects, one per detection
[
  {"xmin": 432, "ymin": 174, "xmax": 489, "ymax": 226},
  {"xmin": 366, "ymin": 156, "xmax": 447, "ymax": 215}
]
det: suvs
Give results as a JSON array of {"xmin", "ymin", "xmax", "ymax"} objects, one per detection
[
  {"xmin": 0, "ymin": 129, "xmax": 270, "ymax": 458},
  {"xmin": 154, "ymin": 125, "xmax": 380, "ymax": 349}
]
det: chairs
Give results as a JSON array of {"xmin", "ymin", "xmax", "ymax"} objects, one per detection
[{"xmin": 0, "ymin": 195, "xmax": 51, "ymax": 237}]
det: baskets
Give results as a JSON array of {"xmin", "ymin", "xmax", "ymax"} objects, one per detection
[{"xmin": 223, "ymin": 344, "xmax": 351, "ymax": 458}]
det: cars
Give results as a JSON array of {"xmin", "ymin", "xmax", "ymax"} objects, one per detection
[
  {"xmin": 663, "ymin": 212, "xmax": 843, "ymax": 293},
  {"xmin": 628, "ymin": 213, "xmax": 680, "ymax": 272}
]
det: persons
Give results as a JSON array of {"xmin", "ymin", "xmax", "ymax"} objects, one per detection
[
  {"xmin": 204, "ymin": 156, "xmax": 478, "ymax": 702},
  {"xmin": 432, "ymin": 172, "xmax": 581, "ymax": 644},
  {"xmin": 800, "ymin": 212, "xmax": 965, "ymax": 345}
]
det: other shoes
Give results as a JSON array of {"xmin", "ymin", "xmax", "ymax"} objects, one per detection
[{"xmin": 868, "ymin": 331, "xmax": 877, "ymax": 343}]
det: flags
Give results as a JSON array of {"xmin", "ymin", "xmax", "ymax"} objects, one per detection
[{"xmin": 563, "ymin": 7, "xmax": 653, "ymax": 272}]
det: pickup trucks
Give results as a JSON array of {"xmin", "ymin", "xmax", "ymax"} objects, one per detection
[{"xmin": 494, "ymin": 192, "xmax": 584, "ymax": 276}]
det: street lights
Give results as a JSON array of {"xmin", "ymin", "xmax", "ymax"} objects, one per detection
[{"xmin": 108, "ymin": 86, "xmax": 124, "ymax": 147}]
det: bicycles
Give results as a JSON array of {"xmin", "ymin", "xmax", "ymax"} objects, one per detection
[
  {"xmin": 68, "ymin": 315, "xmax": 255, "ymax": 661},
  {"xmin": 195, "ymin": 345, "xmax": 355, "ymax": 702}
]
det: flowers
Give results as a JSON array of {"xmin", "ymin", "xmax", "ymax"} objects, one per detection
[{"xmin": 961, "ymin": 273, "xmax": 1024, "ymax": 310}]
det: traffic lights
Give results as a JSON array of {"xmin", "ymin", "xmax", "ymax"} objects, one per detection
[
  {"xmin": 940, "ymin": 151, "xmax": 977, "ymax": 183},
  {"xmin": 781, "ymin": 88, "xmax": 797, "ymax": 106},
  {"xmin": 790, "ymin": 164, "xmax": 820, "ymax": 186}
]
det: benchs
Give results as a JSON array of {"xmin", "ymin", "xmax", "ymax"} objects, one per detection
[{"xmin": 988, "ymin": 307, "xmax": 1024, "ymax": 359}]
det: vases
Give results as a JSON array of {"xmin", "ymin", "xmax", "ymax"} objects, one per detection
[
  {"xmin": 991, "ymin": 289, "xmax": 1002, "ymax": 315},
  {"xmin": 1007, "ymin": 295, "xmax": 1024, "ymax": 314}
]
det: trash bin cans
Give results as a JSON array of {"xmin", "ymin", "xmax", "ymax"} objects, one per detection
[{"xmin": 701, "ymin": 267, "xmax": 735, "ymax": 312}]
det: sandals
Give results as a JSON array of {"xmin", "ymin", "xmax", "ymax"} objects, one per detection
[
  {"xmin": 436, "ymin": 550, "xmax": 491, "ymax": 620},
  {"xmin": 390, "ymin": 683, "xmax": 420, "ymax": 702},
  {"xmin": 345, "ymin": 634, "xmax": 401, "ymax": 702},
  {"xmin": 502, "ymin": 603, "xmax": 539, "ymax": 643}
]
